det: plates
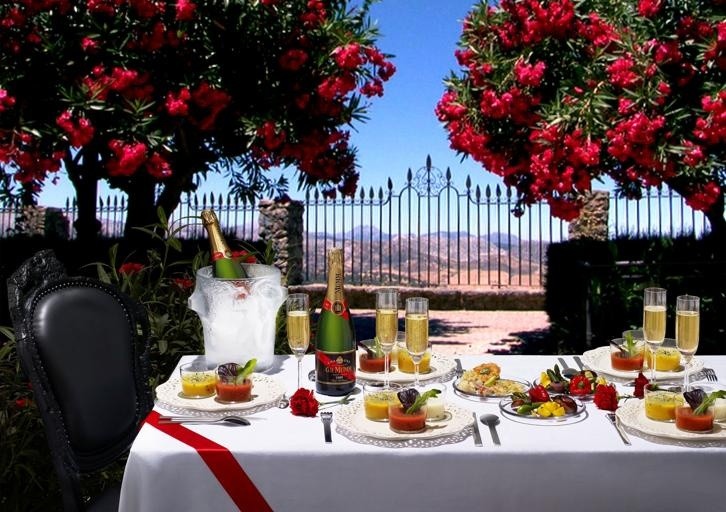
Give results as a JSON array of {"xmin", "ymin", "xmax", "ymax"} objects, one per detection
[
  {"xmin": 615, "ymin": 398, "xmax": 726, "ymax": 440},
  {"xmin": 583, "ymin": 345, "xmax": 705, "ymax": 380},
  {"xmin": 356, "ymin": 355, "xmax": 455, "ymax": 382},
  {"xmin": 534, "ymin": 374, "xmax": 616, "ymax": 399},
  {"xmin": 155, "ymin": 373, "xmax": 284, "ymax": 412},
  {"xmin": 334, "ymin": 397, "xmax": 474, "ymax": 441},
  {"xmin": 499, "ymin": 393, "xmax": 586, "ymax": 420},
  {"xmin": 453, "ymin": 375, "xmax": 533, "ymax": 398}
]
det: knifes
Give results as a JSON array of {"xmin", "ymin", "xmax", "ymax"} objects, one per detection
[{"xmin": 470, "ymin": 411, "xmax": 484, "ymax": 447}]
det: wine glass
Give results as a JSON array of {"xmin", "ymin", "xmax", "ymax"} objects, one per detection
[
  {"xmin": 675, "ymin": 295, "xmax": 700, "ymax": 389},
  {"xmin": 643, "ymin": 287, "xmax": 666, "ymax": 381},
  {"xmin": 286, "ymin": 292, "xmax": 310, "ymax": 390},
  {"xmin": 406, "ymin": 297, "xmax": 429, "ymax": 390},
  {"xmin": 375, "ymin": 288, "xmax": 398, "ymax": 390}
]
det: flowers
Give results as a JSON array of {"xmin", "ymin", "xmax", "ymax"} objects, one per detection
[
  {"xmin": 287, "ymin": 389, "xmax": 354, "ymax": 416},
  {"xmin": 593, "ymin": 383, "xmax": 634, "ymax": 411},
  {"xmin": 634, "ymin": 373, "xmax": 673, "ymax": 399}
]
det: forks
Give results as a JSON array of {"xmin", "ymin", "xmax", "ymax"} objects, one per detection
[
  {"xmin": 703, "ymin": 367, "xmax": 718, "ymax": 382},
  {"xmin": 320, "ymin": 411, "xmax": 335, "ymax": 442},
  {"xmin": 608, "ymin": 413, "xmax": 631, "ymax": 446}
]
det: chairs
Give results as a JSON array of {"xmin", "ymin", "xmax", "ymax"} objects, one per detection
[{"xmin": 5, "ymin": 247, "xmax": 154, "ymax": 512}]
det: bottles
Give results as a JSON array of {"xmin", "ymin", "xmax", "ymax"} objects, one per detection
[
  {"xmin": 201, "ymin": 209, "xmax": 254, "ymax": 298},
  {"xmin": 315, "ymin": 247, "xmax": 356, "ymax": 394}
]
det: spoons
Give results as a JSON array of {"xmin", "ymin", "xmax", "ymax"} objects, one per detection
[
  {"xmin": 480, "ymin": 413, "xmax": 501, "ymax": 445},
  {"xmin": 159, "ymin": 416, "xmax": 251, "ymax": 427}
]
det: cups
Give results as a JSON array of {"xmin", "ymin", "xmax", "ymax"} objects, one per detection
[
  {"xmin": 644, "ymin": 382, "xmax": 685, "ymax": 421},
  {"xmin": 610, "ymin": 339, "xmax": 645, "ymax": 370},
  {"xmin": 180, "ymin": 361, "xmax": 218, "ymax": 399},
  {"xmin": 363, "ymin": 382, "xmax": 402, "ymax": 422},
  {"xmin": 674, "ymin": 391, "xmax": 715, "ymax": 434},
  {"xmin": 388, "ymin": 399, "xmax": 427, "ymax": 434},
  {"xmin": 687, "ymin": 381, "xmax": 726, "ymax": 423},
  {"xmin": 622, "ymin": 330, "xmax": 645, "ymax": 341},
  {"xmin": 648, "ymin": 338, "xmax": 681, "ymax": 371},
  {"xmin": 215, "ymin": 373, "xmax": 254, "ymax": 403}
]
